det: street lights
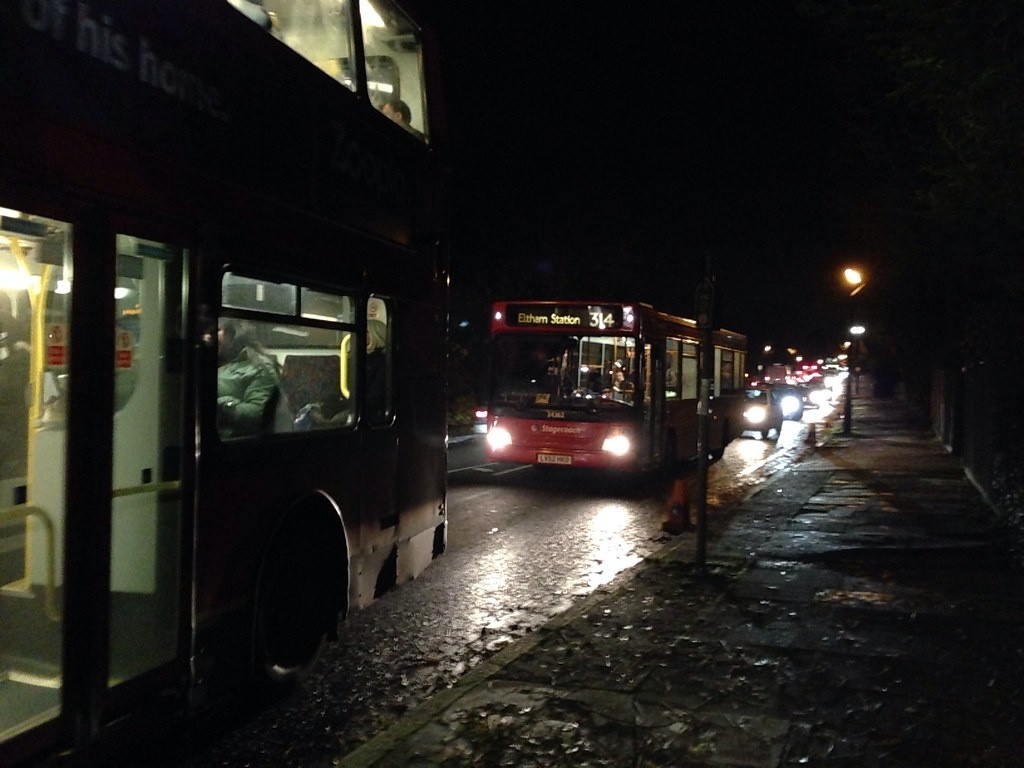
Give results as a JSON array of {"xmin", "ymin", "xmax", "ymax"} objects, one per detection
[
  {"xmin": 842, "ymin": 265, "xmax": 866, "ymax": 437},
  {"xmin": 847, "ymin": 318, "xmax": 860, "ymax": 431}
]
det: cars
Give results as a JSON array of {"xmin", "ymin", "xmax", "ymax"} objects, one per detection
[{"xmin": 744, "ymin": 355, "xmax": 841, "ymax": 440}]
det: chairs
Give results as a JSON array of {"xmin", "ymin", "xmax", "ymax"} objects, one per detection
[{"xmin": 282, "ymin": 355, "xmax": 340, "ymax": 420}]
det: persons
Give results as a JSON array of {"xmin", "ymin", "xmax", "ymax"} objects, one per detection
[
  {"xmin": 217, "ymin": 323, "xmax": 282, "ymax": 433},
  {"xmin": 666, "ymin": 353, "xmax": 677, "ymax": 387},
  {"xmin": 366, "ymin": 319, "xmax": 386, "ymax": 432},
  {"xmin": 0, "ymin": 294, "xmax": 31, "ymax": 477},
  {"xmin": 545, "ymin": 347, "xmax": 636, "ymax": 401},
  {"xmin": 383, "ymin": 101, "xmax": 419, "ymax": 132}
]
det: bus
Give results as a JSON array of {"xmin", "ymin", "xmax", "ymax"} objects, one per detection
[
  {"xmin": 470, "ymin": 297, "xmax": 747, "ymax": 495},
  {"xmin": 2, "ymin": 0, "xmax": 453, "ymax": 768}
]
